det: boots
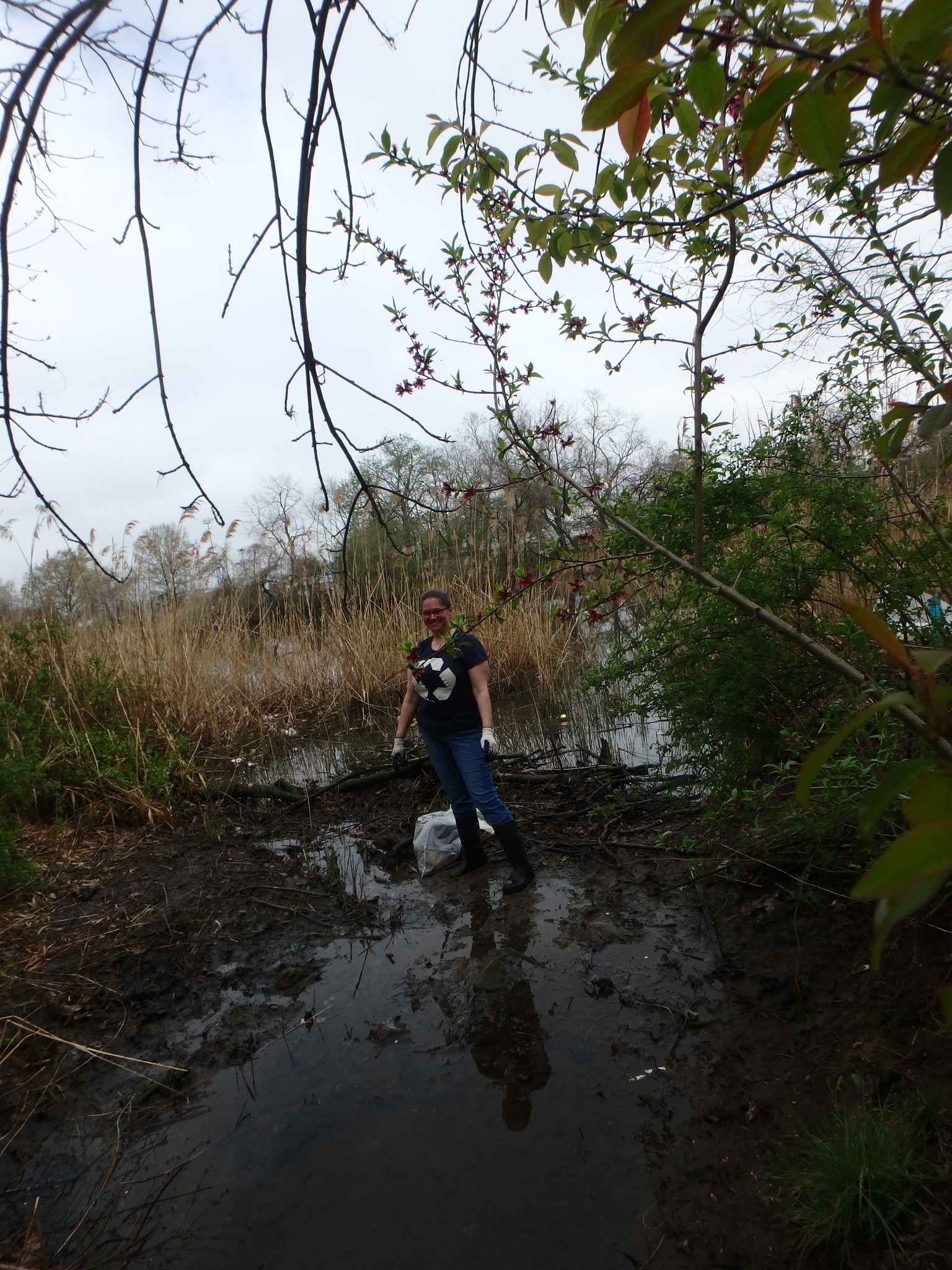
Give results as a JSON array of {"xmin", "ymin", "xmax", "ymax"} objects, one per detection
[
  {"xmin": 492, "ymin": 819, "xmax": 535, "ymax": 893},
  {"xmin": 447, "ymin": 808, "xmax": 488, "ymax": 878}
]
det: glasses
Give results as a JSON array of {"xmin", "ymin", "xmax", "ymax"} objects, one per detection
[{"xmin": 420, "ymin": 607, "xmax": 449, "ymax": 619}]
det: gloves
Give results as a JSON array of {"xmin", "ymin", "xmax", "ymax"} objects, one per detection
[
  {"xmin": 391, "ymin": 737, "xmax": 404, "ymax": 774},
  {"xmin": 480, "ymin": 727, "xmax": 499, "ymax": 762}
]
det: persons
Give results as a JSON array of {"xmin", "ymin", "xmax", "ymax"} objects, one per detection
[{"xmin": 391, "ymin": 590, "xmax": 536, "ymax": 894}]
